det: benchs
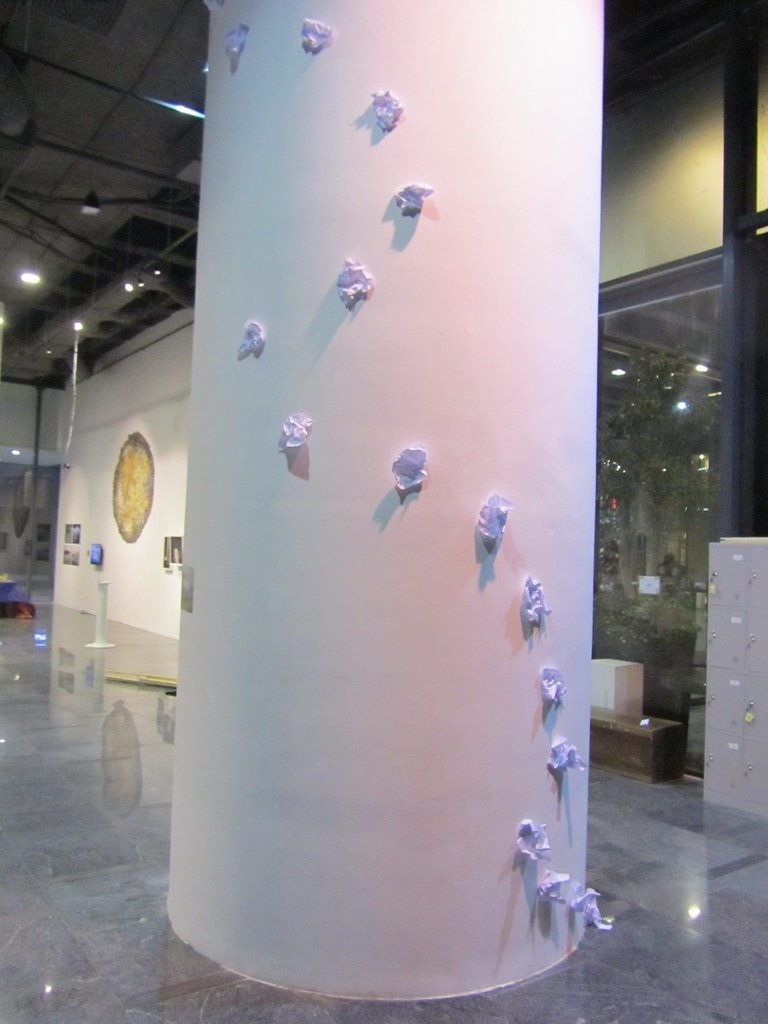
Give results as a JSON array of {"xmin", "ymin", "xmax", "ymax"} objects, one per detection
[{"xmin": 590, "ymin": 704, "xmax": 686, "ymax": 783}]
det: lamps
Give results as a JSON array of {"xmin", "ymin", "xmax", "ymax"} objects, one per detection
[{"xmin": 79, "ymin": 163, "xmax": 102, "ymax": 216}]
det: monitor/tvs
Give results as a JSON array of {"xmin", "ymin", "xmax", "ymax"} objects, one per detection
[{"xmin": 91, "ymin": 544, "xmax": 103, "ymax": 564}]
